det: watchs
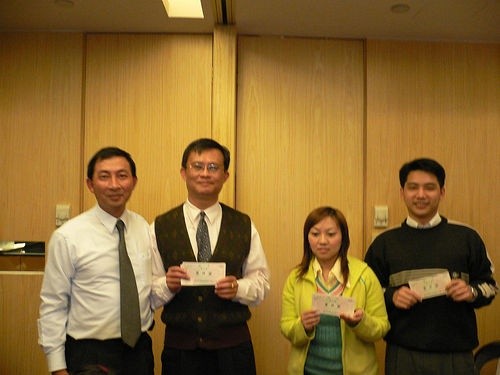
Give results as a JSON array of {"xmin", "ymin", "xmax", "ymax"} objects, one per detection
[{"xmin": 466, "ymin": 284, "xmax": 476, "ymax": 303}]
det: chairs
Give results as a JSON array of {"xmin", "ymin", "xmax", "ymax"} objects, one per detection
[{"xmin": 474, "ymin": 340, "xmax": 500, "ymax": 375}]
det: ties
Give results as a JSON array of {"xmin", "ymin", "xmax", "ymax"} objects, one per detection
[
  {"xmin": 116, "ymin": 220, "xmax": 142, "ymax": 347},
  {"xmin": 196, "ymin": 211, "xmax": 212, "ymax": 262}
]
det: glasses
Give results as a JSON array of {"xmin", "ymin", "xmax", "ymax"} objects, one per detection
[{"xmin": 186, "ymin": 163, "xmax": 224, "ymax": 172}]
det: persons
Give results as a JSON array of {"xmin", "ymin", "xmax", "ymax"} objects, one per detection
[
  {"xmin": 279, "ymin": 206, "xmax": 391, "ymax": 375},
  {"xmin": 146, "ymin": 138, "xmax": 270, "ymax": 375},
  {"xmin": 364, "ymin": 158, "xmax": 499, "ymax": 375},
  {"xmin": 37, "ymin": 146, "xmax": 155, "ymax": 375}
]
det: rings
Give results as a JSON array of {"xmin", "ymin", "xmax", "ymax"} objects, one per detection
[{"xmin": 231, "ymin": 282, "xmax": 235, "ymax": 289}]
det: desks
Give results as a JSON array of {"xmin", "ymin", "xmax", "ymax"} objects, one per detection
[
  {"xmin": 0, "ymin": 252, "xmax": 45, "ymax": 271},
  {"xmin": 0, "ymin": 271, "xmax": 52, "ymax": 375}
]
